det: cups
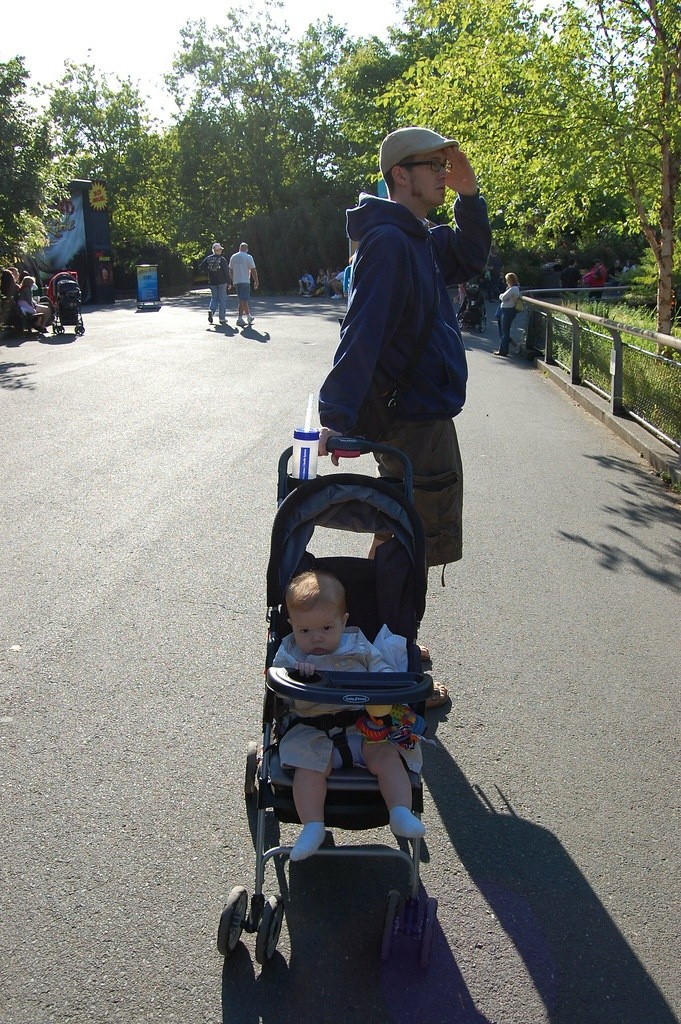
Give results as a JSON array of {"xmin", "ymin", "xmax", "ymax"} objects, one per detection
[{"xmin": 292, "ymin": 426, "xmax": 320, "ymax": 479}]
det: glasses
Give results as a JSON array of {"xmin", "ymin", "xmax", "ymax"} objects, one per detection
[{"xmin": 398, "ymin": 160, "xmax": 452, "ymax": 174}]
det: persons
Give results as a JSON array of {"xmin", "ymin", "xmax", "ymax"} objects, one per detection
[
  {"xmin": 273, "ymin": 571, "xmax": 427, "ymax": 860},
  {"xmin": 198, "ymin": 243, "xmax": 233, "ymax": 323},
  {"xmin": 316, "ymin": 127, "xmax": 491, "ymax": 708},
  {"xmin": 298, "ymin": 254, "xmax": 353, "ymax": 299},
  {"xmin": 454, "ymin": 279, "xmax": 481, "ymax": 309},
  {"xmin": 493, "ymin": 271, "xmax": 522, "ymax": 356},
  {"xmin": 560, "ymin": 258, "xmax": 607, "ymax": 303},
  {"xmin": 0, "ymin": 268, "xmax": 52, "ymax": 333},
  {"xmin": 608, "ymin": 259, "xmax": 636, "ymax": 294},
  {"xmin": 228, "ymin": 243, "xmax": 259, "ymax": 326}
]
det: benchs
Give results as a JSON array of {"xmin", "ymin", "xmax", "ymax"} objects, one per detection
[{"xmin": 15, "ymin": 295, "xmax": 44, "ymax": 336}]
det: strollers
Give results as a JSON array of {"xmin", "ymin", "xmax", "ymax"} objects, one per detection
[
  {"xmin": 50, "ymin": 271, "xmax": 86, "ymax": 337},
  {"xmin": 456, "ymin": 285, "xmax": 488, "ymax": 333},
  {"xmin": 216, "ymin": 435, "xmax": 441, "ymax": 973}
]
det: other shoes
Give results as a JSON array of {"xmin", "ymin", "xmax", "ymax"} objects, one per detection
[
  {"xmin": 427, "ymin": 681, "xmax": 447, "ymax": 707},
  {"xmin": 330, "ymin": 294, "xmax": 342, "ymax": 300},
  {"xmin": 219, "ymin": 319, "xmax": 227, "ymax": 323},
  {"xmin": 34, "ymin": 324, "xmax": 48, "ymax": 333},
  {"xmin": 207, "ymin": 311, "xmax": 213, "ymax": 323},
  {"xmin": 493, "ymin": 347, "xmax": 508, "ymax": 357},
  {"xmin": 246, "ymin": 314, "xmax": 253, "ymax": 322},
  {"xmin": 235, "ymin": 319, "xmax": 245, "ymax": 326}
]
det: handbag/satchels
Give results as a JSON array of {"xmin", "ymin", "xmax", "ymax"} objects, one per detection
[
  {"xmin": 515, "ymin": 296, "xmax": 529, "ymax": 312},
  {"xmin": 358, "ymin": 373, "xmax": 399, "ymax": 427}
]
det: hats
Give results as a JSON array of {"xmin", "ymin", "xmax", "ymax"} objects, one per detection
[
  {"xmin": 211, "ymin": 243, "xmax": 224, "ymax": 252},
  {"xmin": 380, "ymin": 127, "xmax": 460, "ymax": 177}
]
